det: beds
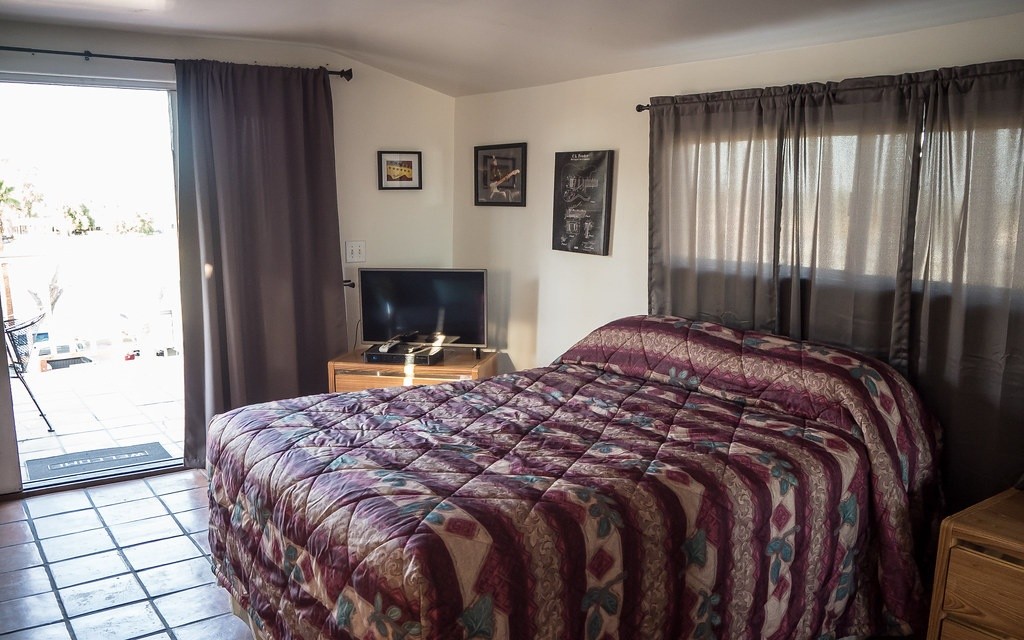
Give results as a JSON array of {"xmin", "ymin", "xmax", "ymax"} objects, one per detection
[{"xmin": 206, "ymin": 314, "xmax": 932, "ymax": 640}]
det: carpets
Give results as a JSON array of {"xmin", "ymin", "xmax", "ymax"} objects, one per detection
[{"xmin": 23, "ymin": 441, "xmax": 173, "ymax": 480}]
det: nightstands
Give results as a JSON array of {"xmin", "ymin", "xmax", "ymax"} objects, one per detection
[
  {"xmin": 927, "ymin": 488, "xmax": 1024, "ymax": 640},
  {"xmin": 328, "ymin": 346, "xmax": 498, "ymax": 394}
]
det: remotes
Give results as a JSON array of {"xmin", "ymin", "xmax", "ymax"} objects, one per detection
[{"xmin": 379, "ymin": 340, "xmax": 400, "ymax": 352}]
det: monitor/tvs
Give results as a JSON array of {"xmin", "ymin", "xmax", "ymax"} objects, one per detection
[{"xmin": 358, "ymin": 268, "xmax": 488, "ymax": 359}]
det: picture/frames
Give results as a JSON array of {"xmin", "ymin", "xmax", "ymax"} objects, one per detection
[
  {"xmin": 377, "ymin": 150, "xmax": 422, "ymax": 191},
  {"xmin": 474, "ymin": 142, "xmax": 528, "ymax": 207}
]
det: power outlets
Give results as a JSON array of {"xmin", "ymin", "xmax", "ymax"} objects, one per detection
[{"xmin": 345, "ymin": 240, "xmax": 366, "ymax": 262}]
